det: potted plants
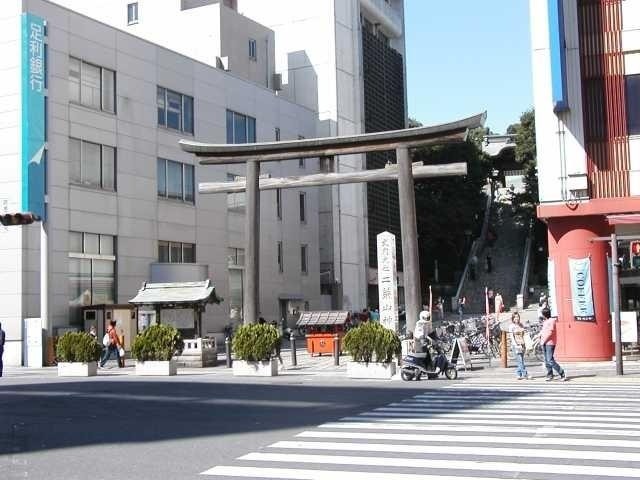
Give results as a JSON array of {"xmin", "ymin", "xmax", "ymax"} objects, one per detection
[
  {"xmin": 55, "ymin": 331, "xmax": 102, "ymax": 376},
  {"xmin": 130, "ymin": 325, "xmax": 183, "ymax": 376},
  {"xmin": 232, "ymin": 321, "xmax": 282, "ymax": 377},
  {"xmin": 341, "ymin": 321, "xmax": 402, "ymax": 380}
]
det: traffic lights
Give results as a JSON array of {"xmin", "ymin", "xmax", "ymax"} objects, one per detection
[
  {"xmin": 0, "ymin": 212, "xmax": 32, "ymax": 226},
  {"xmin": 630, "ymin": 240, "xmax": 640, "ymax": 268}
]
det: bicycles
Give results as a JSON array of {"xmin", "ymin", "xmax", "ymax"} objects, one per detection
[{"xmin": 402, "ymin": 315, "xmax": 545, "ymax": 362}]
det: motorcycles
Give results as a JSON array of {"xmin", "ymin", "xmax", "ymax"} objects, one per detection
[{"xmin": 400, "ymin": 340, "xmax": 458, "ymax": 381}]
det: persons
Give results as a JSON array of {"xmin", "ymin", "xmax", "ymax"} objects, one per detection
[
  {"xmin": 436, "ymin": 252, "xmax": 503, "ymax": 319},
  {"xmin": 360, "ymin": 307, "xmax": 379, "ymax": 322},
  {"xmin": 99, "ymin": 319, "xmax": 122, "ymax": 369},
  {"xmin": 90, "ymin": 325, "xmax": 97, "ymax": 339},
  {"xmin": 399, "ymin": 306, "xmax": 405, "ymax": 320},
  {"xmin": 533, "ymin": 308, "xmax": 566, "ymax": 382},
  {"xmin": 270, "ymin": 320, "xmax": 283, "ymax": 365},
  {"xmin": 509, "ymin": 312, "xmax": 531, "ymax": 379},
  {"xmin": 412, "ymin": 311, "xmax": 438, "ymax": 380},
  {"xmin": 537, "ymin": 292, "xmax": 548, "ymax": 320},
  {"xmin": 0, "ymin": 323, "xmax": 5, "ymax": 377}
]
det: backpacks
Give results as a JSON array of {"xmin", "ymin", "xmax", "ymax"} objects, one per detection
[{"xmin": 103, "ymin": 327, "xmax": 111, "ymax": 346}]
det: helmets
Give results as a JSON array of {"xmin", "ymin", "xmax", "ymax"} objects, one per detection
[{"xmin": 420, "ymin": 310, "xmax": 431, "ymax": 324}]
